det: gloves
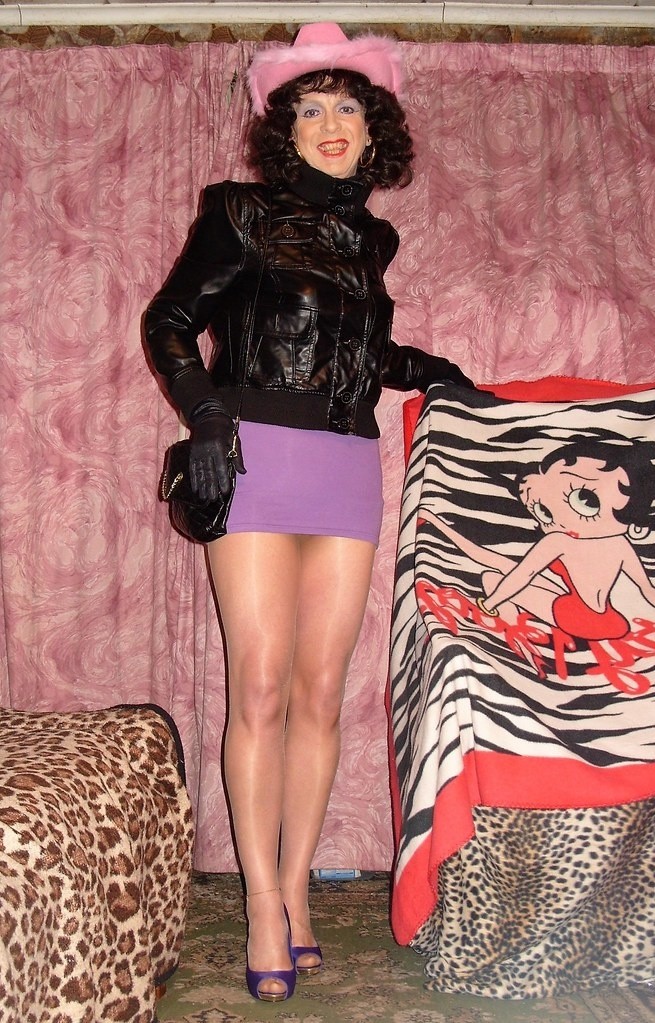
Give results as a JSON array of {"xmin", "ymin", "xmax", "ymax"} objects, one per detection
[
  {"xmin": 449, "ymin": 360, "xmax": 475, "ymax": 392},
  {"xmin": 187, "ymin": 393, "xmax": 247, "ymax": 501}
]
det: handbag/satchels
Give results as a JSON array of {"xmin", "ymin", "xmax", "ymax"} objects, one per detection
[{"xmin": 157, "ymin": 439, "xmax": 237, "ymax": 545}]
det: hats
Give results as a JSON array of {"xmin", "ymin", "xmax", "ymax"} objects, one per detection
[{"xmin": 243, "ymin": 22, "xmax": 404, "ymax": 119}]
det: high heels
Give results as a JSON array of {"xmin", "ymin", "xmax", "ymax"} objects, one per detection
[
  {"xmin": 285, "ymin": 908, "xmax": 322, "ymax": 975},
  {"xmin": 246, "ymin": 916, "xmax": 297, "ymax": 1002}
]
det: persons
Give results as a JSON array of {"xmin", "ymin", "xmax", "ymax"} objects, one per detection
[{"xmin": 144, "ymin": 23, "xmax": 474, "ymax": 1002}]
